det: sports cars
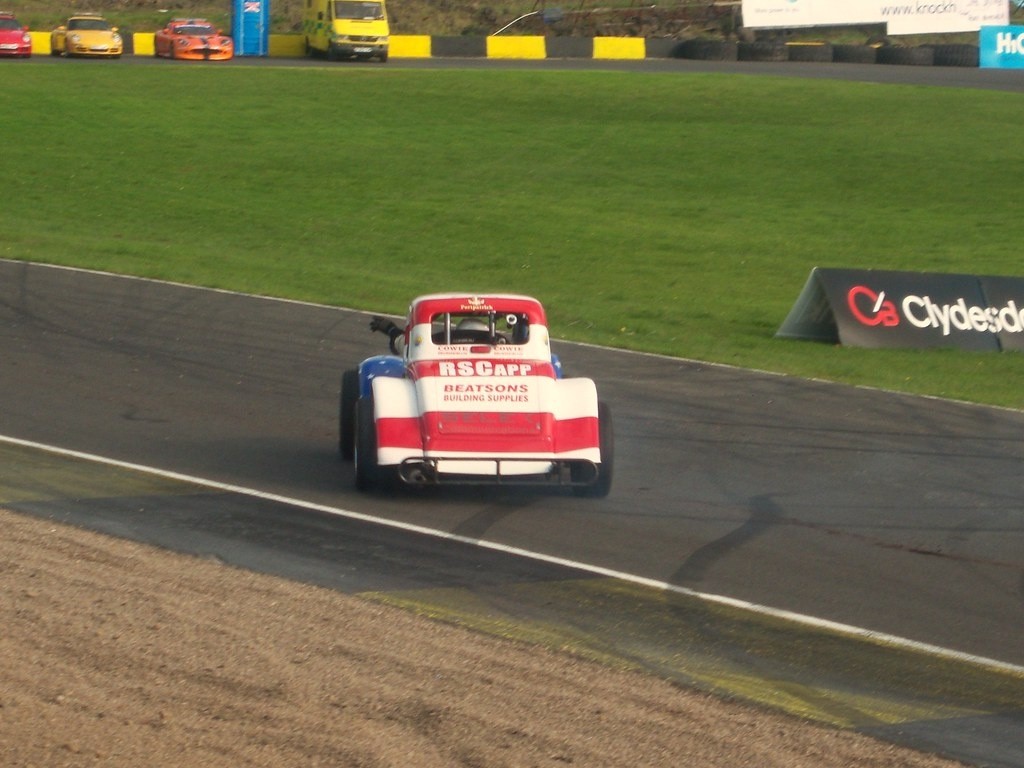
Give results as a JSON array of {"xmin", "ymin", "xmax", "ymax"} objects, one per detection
[
  {"xmin": 49, "ymin": 15, "xmax": 122, "ymax": 58},
  {"xmin": 154, "ymin": 20, "xmax": 234, "ymax": 61}
]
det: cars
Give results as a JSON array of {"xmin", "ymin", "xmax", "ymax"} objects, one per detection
[
  {"xmin": 0, "ymin": 11, "xmax": 31, "ymax": 58},
  {"xmin": 338, "ymin": 291, "xmax": 604, "ymax": 496}
]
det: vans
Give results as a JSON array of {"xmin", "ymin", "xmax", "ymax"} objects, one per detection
[{"xmin": 303, "ymin": 0, "xmax": 389, "ymax": 61}]
